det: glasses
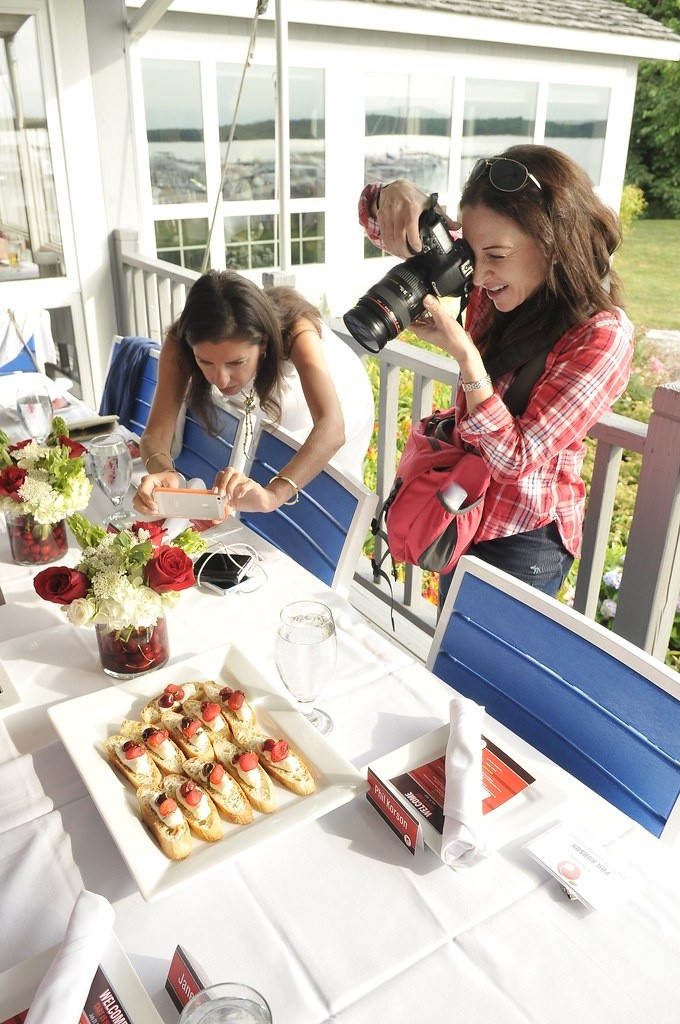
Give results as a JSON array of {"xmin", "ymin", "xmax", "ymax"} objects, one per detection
[{"xmin": 463, "ymin": 157, "xmax": 544, "ymax": 198}]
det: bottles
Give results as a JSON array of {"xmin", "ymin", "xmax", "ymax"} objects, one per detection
[{"xmin": 442, "ymin": 481, "xmax": 468, "ymax": 511}]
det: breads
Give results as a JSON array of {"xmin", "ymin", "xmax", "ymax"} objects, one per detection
[{"xmin": 104, "ymin": 680, "xmax": 317, "ymax": 861}]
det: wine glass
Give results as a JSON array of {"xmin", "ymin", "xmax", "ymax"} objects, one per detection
[
  {"xmin": 274, "ymin": 599, "xmax": 338, "ymax": 737},
  {"xmin": 88, "ymin": 432, "xmax": 139, "ymax": 530},
  {"xmin": 17, "ymin": 384, "xmax": 54, "ymax": 446}
]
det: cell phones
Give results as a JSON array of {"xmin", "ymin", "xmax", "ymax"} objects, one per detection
[
  {"xmin": 199, "ymin": 574, "xmax": 251, "ymax": 595},
  {"xmin": 153, "ymin": 488, "xmax": 229, "ymax": 522}
]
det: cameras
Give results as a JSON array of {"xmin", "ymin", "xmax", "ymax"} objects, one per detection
[{"xmin": 344, "ymin": 210, "xmax": 474, "ymax": 354}]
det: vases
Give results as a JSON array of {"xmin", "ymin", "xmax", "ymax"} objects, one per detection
[
  {"xmin": 97, "ymin": 614, "xmax": 169, "ymax": 679},
  {"xmin": 4, "ymin": 510, "xmax": 67, "ymax": 566}
]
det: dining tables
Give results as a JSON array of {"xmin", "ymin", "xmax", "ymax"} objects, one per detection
[{"xmin": 0, "ymin": 374, "xmax": 680, "ymax": 1024}]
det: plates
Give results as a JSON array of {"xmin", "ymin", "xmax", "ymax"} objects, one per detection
[{"xmin": 46, "ymin": 642, "xmax": 372, "ymax": 905}]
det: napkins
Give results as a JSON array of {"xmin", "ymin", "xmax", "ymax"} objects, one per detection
[
  {"xmin": 23, "ymin": 889, "xmax": 114, "ymax": 1023},
  {"xmin": 159, "ymin": 476, "xmax": 205, "ymax": 544},
  {"xmin": 85, "ymin": 424, "xmax": 130, "ymax": 467},
  {"xmin": 440, "ymin": 695, "xmax": 482, "ymax": 865},
  {"xmin": 6, "ymin": 378, "xmax": 73, "ymax": 419}
]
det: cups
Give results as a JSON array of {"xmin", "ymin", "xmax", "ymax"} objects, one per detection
[{"xmin": 177, "ymin": 981, "xmax": 273, "ymax": 1024}]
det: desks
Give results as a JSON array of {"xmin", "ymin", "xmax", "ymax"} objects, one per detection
[{"xmin": 0, "ymin": 261, "xmax": 40, "ymax": 282}]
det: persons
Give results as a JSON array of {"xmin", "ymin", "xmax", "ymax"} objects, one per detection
[
  {"xmin": 133, "ymin": 269, "xmax": 376, "ymax": 525},
  {"xmin": 358, "ymin": 143, "xmax": 635, "ymax": 629}
]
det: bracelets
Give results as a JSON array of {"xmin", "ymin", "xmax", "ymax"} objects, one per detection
[
  {"xmin": 164, "ymin": 470, "xmax": 185, "ymax": 480},
  {"xmin": 269, "ymin": 476, "xmax": 299, "ymax": 506},
  {"xmin": 376, "ymin": 184, "xmax": 389, "ymax": 210},
  {"xmin": 145, "ymin": 452, "xmax": 175, "ymax": 469},
  {"xmin": 461, "ymin": 373, "xmax": 492, "ymax": 392}
]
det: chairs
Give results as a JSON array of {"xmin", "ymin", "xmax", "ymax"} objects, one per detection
[
  {"xmin": 0, "ymin": 308, "xmax": 57, "ymax": 378},
  {"xmin": 228, "ymin": 419, "xmax": 380, "ymax": 597},
  {"xmin": 105, "ymin": 336, "xmax": 194, "ymax": 463},
  {"xmin": 168, "ymin": 380, "xmax": 257, "ymax": 489},
  {"xmin": 425, "ymin": 554, "xmax": 680, "ymax": 838}
]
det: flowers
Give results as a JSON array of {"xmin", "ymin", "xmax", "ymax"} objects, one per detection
[
  {"xmin": 34, "ymin": 512, "xmax": 206, "ymax": 646},
  {"xmin": 0, "ymin": 418, "xmax": 94, "ymax": 533}
]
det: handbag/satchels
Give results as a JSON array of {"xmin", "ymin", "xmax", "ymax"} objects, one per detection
[{"xmin": 372, "ymin": 407, "xmax": 492, "ymax": 575}]
